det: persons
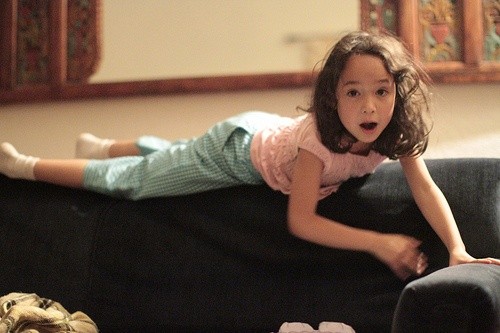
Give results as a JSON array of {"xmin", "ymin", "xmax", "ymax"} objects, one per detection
[{"xmin": 1, "ymin": 31, "xmax": 495, "ymax": 280}]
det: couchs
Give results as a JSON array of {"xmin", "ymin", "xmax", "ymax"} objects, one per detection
[{"xmin": 0, "ymin": 158, "xmax": 500, "ymax": 333}]
